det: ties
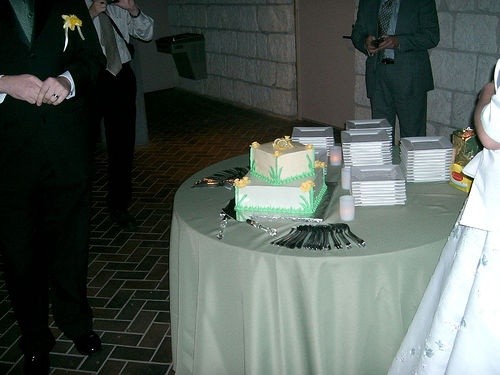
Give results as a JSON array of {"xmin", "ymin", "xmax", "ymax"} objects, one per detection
[
  {"xmin": 98, "ymin": 9, "xmax": 123, "ymax": 76},
  {"xmin": 378, "ymin": 0, "xmax": 393, "ymax": 46}
]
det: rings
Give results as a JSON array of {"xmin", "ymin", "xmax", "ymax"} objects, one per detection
[{"xmin": 53, "ymin": 94, "xmax": 59, "ymax": 99}]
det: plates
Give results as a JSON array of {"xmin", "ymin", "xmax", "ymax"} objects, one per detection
[{"xmin": 290, "ymin": 117, "xmax": 455, "ymax": 207}]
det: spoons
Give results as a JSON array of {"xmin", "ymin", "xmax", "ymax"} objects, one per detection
[{"xmin": 270, "ymin": 221, "xmax": 366, "ymax": 250}]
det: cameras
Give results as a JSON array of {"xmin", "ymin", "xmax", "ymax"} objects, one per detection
[
  {"xmin": 106, "ymin": 0, "xmax": 119, "ymax": 4},
  {"xmin": 372, "ymin": 39, "xmax": 384, "ymax": 48}
]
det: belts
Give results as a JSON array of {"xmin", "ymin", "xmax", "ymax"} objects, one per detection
[{"xmin": 380, "ymin": 58, "xmax": 394, "ymax": 65}]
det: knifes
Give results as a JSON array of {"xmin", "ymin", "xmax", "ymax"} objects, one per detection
[
  {"xmin": 221, "ymin": 208, "xmax": 277, "ymax": 235},
  {"xmin": 214, "ymin": 199, "xmax": 232, "ymax": 239}
]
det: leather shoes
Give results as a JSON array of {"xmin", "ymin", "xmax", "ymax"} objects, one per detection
[
  {"xmin": 73, "ymin": 330, "xmax": 101, "ymax": 355},
  {"xmin": 23, "ymin": 348, "xmax": 50, "ymax": 375}
]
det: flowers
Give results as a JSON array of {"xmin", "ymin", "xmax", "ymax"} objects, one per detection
[{"xmin": 62, "ymin": 15, "xmax": 85, "ymax": 52}]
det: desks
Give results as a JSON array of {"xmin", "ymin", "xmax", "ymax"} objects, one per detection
[{"xmin": 169, "ymin": 145, "xmax": 468, "ymax": 375}]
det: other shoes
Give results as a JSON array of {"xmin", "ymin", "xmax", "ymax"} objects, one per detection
[{"xmin": 110, "ymin": 209, "xmax": 137, "ymax": 230}]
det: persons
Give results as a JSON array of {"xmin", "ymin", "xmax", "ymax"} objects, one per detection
[
  {"xmin": 351, "ymin": 0, "xmax": 441, "ymax": 146},
  {"xmin": 85, "ymin": 0, "xmax": 155, "ymax": 230},
  {"xmin": 0, "ymin": 0, "xmax": 108, "ymax": 375},
  {"xmin": 388, "ymin": 58, "xmax": 500, "ymax": 375}
]
